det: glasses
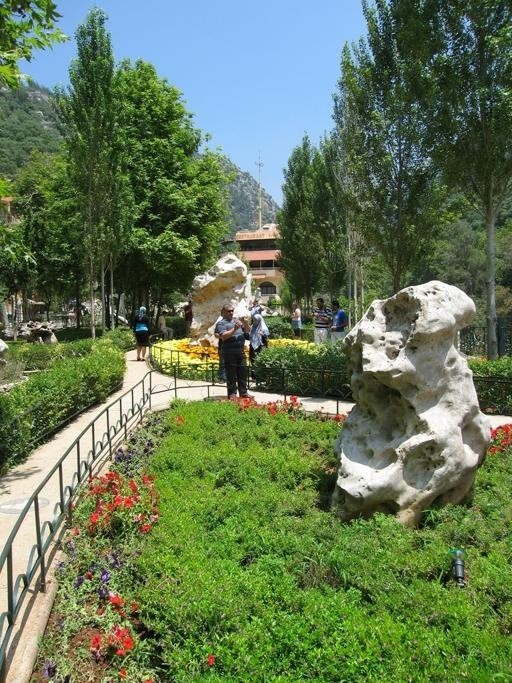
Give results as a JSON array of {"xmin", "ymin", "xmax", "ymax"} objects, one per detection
[{"xmin": 228, "ymin": 310, "xmax": 233, "ymax": 313}]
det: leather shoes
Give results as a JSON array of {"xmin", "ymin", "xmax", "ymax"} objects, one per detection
[
  {"xmin": 239, "ymin": 393, "xmax": 255, "ymax": 398},
  {"xmin": 228, "ymin": 393, "xmax": 236, "ymax": 400}
]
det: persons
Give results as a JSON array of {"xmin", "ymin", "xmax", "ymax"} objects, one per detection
[
  {"xmin": 291, "ymin": 303, "xmax": 301, "ymax": 340},
  {"xmin": 158, "ymin": 311, "xmax": 173, "ymax": 341},
  {"xmin": 251, "ymin": 299, "xmax": 264, "ymax": 321},
  {"xmin": 312, "ymin": 298, "xmax": 332, "ymax": 343},
  {"xmin": 244, "ymin": 315, "xmax": 269, "ymax": 382},
  {"xmin": 214, "ymin": 309, "xmax": 226, "ymax": 382},
  {"xmin": 184, "ymin": 301, "xmax": 192, "ymax": 339},
  {"xmin": 218, "ymin": 304, "xmax": 254, "ymax": 400},
  {"xmin": 330, "ymin": 300, "xmax": 348, "ymax": 343},
  {"xmin": 133, "ymin": 306, "xmax": 150, "ymax": 361}
]
